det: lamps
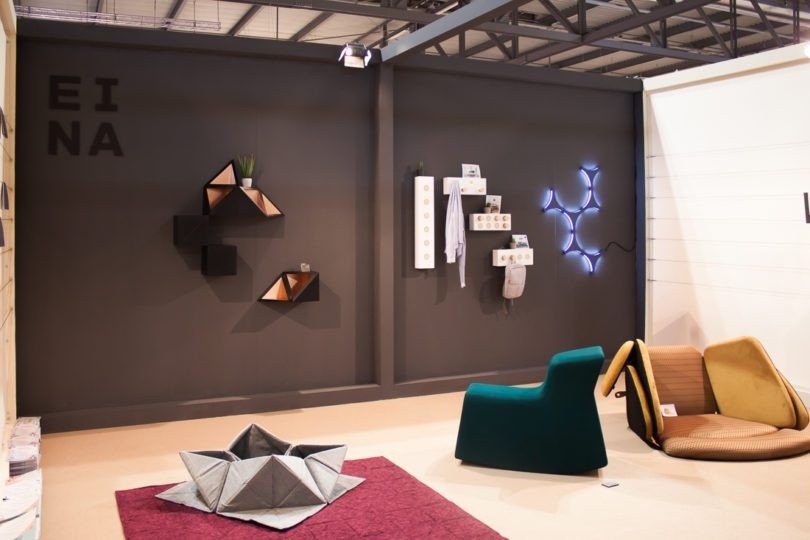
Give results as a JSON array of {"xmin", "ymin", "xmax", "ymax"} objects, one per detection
[{"xmin": 336, "ymin": 41, "xmax": 372, "ymax": 69}]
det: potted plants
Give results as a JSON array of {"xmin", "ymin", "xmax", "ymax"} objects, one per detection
[{"xmin": 238, "ymin": 155, "xmax": 256, "ymax": 188}]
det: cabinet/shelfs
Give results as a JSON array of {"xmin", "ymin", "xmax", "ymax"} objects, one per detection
[
  {"xmin": 414, "ymin": 163, "xmax": 533, "ymax": 268},
  {"xmin": 173, "ymin": 159, "xmax": 320, "ymax": 303}
]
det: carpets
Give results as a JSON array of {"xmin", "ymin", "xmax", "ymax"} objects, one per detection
[{"xmin": 114, "ymin": 455, "xmax": 510, "ymax": 540}]
